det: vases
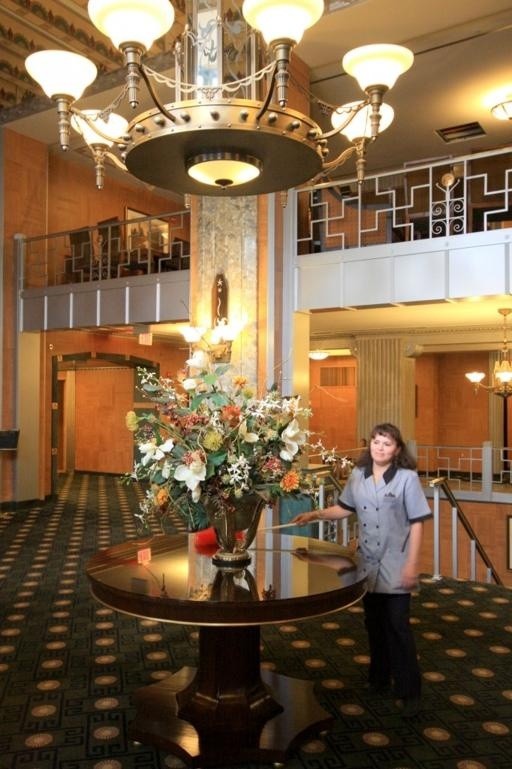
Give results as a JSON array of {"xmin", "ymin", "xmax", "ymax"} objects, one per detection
[{"xmin": 210, "ymin": 492, "xmax": 266, "ymax": 571}]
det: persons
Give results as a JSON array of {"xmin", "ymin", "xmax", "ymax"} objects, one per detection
[
  {"xmin": 354, "ymin": 438, "xmax": 370, "ymax": 467},
  {"xmin": 290, "ymin": 422, "xmax": 436, "ymax": 717}
]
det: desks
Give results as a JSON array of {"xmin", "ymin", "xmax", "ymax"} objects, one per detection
[
  {"xmin": 85, "ymin": 533, "xmax": 369, "ymax": 767},
  {"xmin": 129, "ymin": 247, "xmax": 167, "ymax": 274},
  {"xmin": 409, "ymin": 208, "xmax": 455, "ymax": 238}
]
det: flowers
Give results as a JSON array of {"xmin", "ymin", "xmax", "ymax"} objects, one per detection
[{"xmin": 115, "ymin": 325, "xmax": 355, "ymax": 535}]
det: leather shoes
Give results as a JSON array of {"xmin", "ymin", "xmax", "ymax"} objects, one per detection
[{"xmin": 400, "ymin": 698, "xmax": 424, "ymax": 716}]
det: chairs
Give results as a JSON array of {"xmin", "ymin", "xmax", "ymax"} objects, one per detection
[
  {"xmin": 70, "ymin": 226, "xmax": 100, "ymax": 281},
  {"xmin": 468, "ymin": 143, "xmax": 512, "ymax": 233},
  {"xmin": 164, "ymin": 237, "xmax": 190, "ymax": 270},
  {"xmin": 98, "ymin": 216, "xmax": 123, "ymax": 267},
  {"xmin": 403, "ymin": 154, "xmax": 454, "ymax": 241},
  {"xmin": 317, "ymin": 172, "xmax": 392, "ymax": 252}
]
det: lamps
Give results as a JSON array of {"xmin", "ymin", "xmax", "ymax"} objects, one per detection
[
  {"xmin": 490, "ymin": 100, "xmax": 512, "ymax": 121},
  {"xmin": 178, "ymin": 319, "xmax": 243, "ymax": 360},
  {"xmin": 25, "ymin": 0, "xmax": 414, "ymax": 197},
  {"xmin": 465, "ymin": 308, "xmax": 512, "ymax": 398}
]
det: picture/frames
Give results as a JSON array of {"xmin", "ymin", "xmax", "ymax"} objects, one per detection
[{"xmin": 124, "ymin": 205, "xmax": 172, "ymax": 254}]
